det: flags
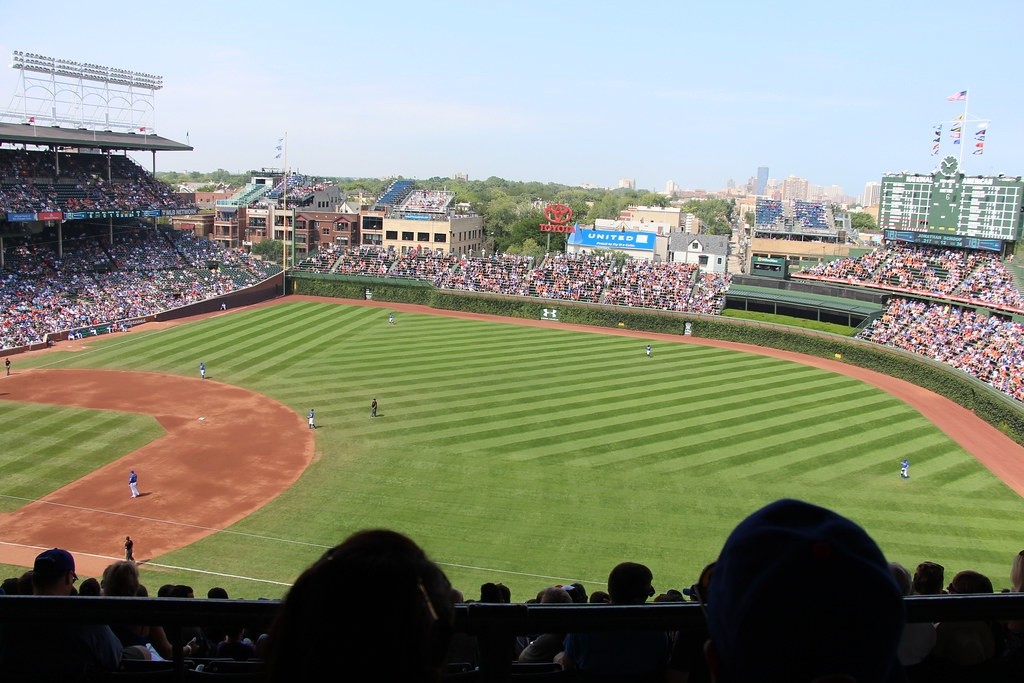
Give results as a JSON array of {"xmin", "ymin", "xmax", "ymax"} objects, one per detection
[
  {"xmin": 930, "ymin": 150, "xmax": 938, "ymax": 156},
  {"xmin": 953, "ymin": 139, "xmax": 960, "ymax": 144},
  {"xmin": 948, "ymin": 133, "xmax": 960, "ymax": 138},
  {"xmin": 946, "ymin": 90, "xmax": 966, "ymax": 102},
  {"xmin": 932, "ymin": 143, "xmax": 939, "ymax": 149},
  {"xmin": 953, "ymin": 122, "xmax": 961, "ymax": 126},
  {"xmin": 978, "ymin": 123, "xmax": 987, "ymax": 127},
  {"xmin": 976, "ymin": 142, "xmax": 984, "ymax": 147},
  {"xmin": 974, "ymin": 136, "xmax": 985, "ymax": 140},
  {"xmin": 954, "ymin": 114, "xmax": 964, "ymax": 120},
  {"xmin": 972, "ymin": 149, "xmax": 983, "ymax": 155},
  {"xmin": 934, "ymin": 130, "xmax": 941, "ymax": 136},
  {"xmin": 932, "ymin": 137, "xmax": 940, "ymax": 143},
  {"xmin": 949, "ymin": 127, "xmax": 961, "ymax": 132},
  {"xmin": 932, "ymin": 123, "xmax": 942, "ymax": 129},
  {"xmin": 975, "ymin": 129, "xmax": 986, "ymax": 135}
]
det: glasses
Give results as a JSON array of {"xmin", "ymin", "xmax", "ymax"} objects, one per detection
[
  {"xmin": 73, "ymin": 579, "xmax": 76, "ymax": 583},
  {"xmin": 949, "ymin": 583, "xmax": 959, "ymax": 593},
  {"xmin": 644, "ymin": 585, "xmax": 655, "ymax": 597},
  {"xmin": 101, "ymin": 580, "xmax": 107, "ymax": 589}
]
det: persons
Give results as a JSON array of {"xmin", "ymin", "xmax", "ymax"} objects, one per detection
[
  {"xmin": 292, "ymin": 238, "xmax": 733, "ymax": 315},
  {"xmin": 855, "ymin": 294, "xmax": 1024, "ymax": 409},
  {"xmin": 0, "ymin": 497, "xmax": 1024, "ymax": 683},
  {"xmin": 5, "ymin": 358, "xmax": 11, "ymax": 376},
  {"xmin": 753, "ymin": 255, "xmax": 785, "ymax": 272},
  {"xmin": 389, "ymin": 312, "xmax": 393, "ymax": 322},
  {"xmin": 128, "ymin": 469, "xmax": 140, "ymax": 499},
  {"xmin": 0, "ymin": 119, "xmax": 283, "ymax": 355},
  {"xmin": 199, "ymin": 362, "xmax": 206, "ymax": 379},
  {"xmin": 899, "ymin": 459, "xmax": 910, "ymax": 478},
  {"xmin": 753, "ymin": 195, "xmax": 836, "ymax": 243},
  {"xmin": 370, "ymin": 398, "xmax": 378, "ymax": 417},
  {"xmin": 306, "ymin": 408, "xmax": 317, "ymax": 430},
  {"xmin": 210, "ymin": 169, "xmax": 462, "ymax": 267},
  {"xmin": 790, "ymin": 239, "xmax": 1024, "ymax": 311}
]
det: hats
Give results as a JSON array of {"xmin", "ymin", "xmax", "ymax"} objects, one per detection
[
  {"xmin": 683, "ymin": 585, "xmax": 698, "ymax": 597},
  {"xmin": 560, "ymin": 585, "xmax": 585, "ymax": 603},
  {"xmin": 481, "ymin": 582, "xmax": 502, "ymax": 603},
  {"xmin": 706, "ymin": 499, "xmax": 905, "ymax": 668},
  {"xmin": 951, "ymin": 570, "xmax": 993, "ymax": 594},
  {"xmin": 916, "ymin": 561, "xmax": 944, "ymax": 577},
  {"xmin": 34, "ymin": 547, "xmax": 78, "ymax": 581}
]
